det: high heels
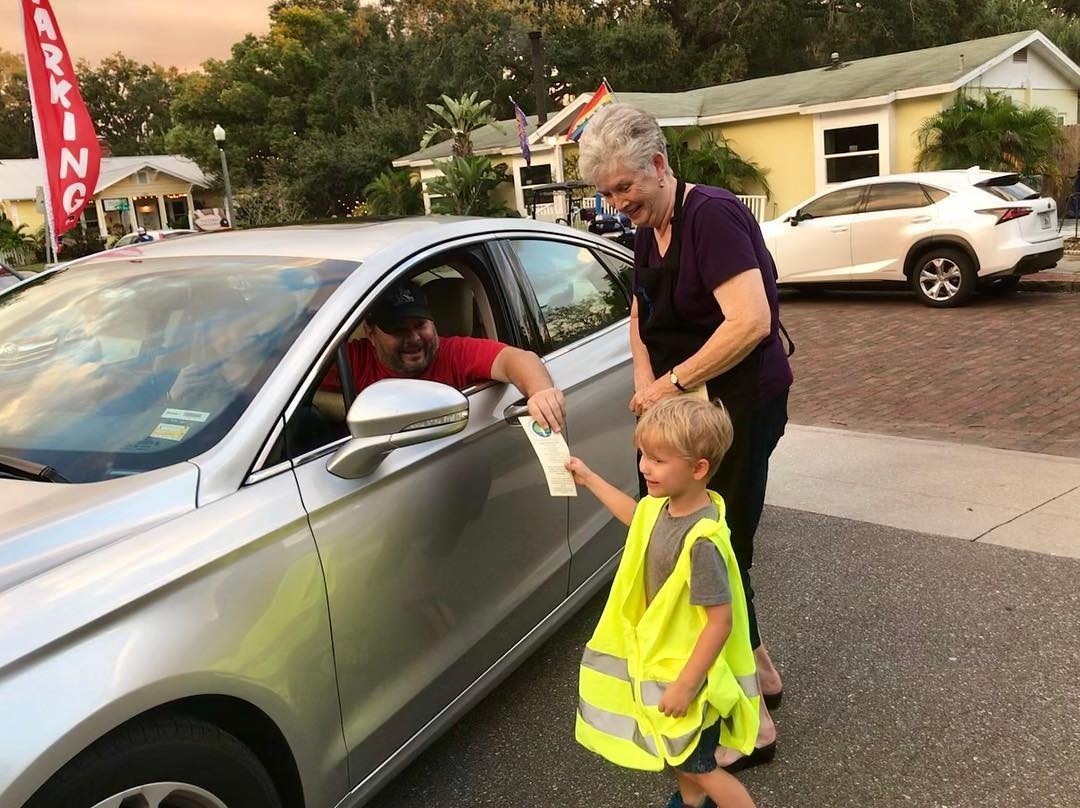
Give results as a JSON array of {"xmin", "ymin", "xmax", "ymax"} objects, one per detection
[
  {"xmin": 714, "ymin": 740, "xmax": 780, "ymax": 772},
  {"xmin": 764, "ymin": 690, "xmax": 783, "ymax": 710}
]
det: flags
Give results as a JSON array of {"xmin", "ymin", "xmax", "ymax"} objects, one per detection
[
  {"xmin": 566, "ymin": 82, "xmax": 614, "ymax": 143},
  {"xmin": 513, "ymin": 102, "xmax": 530, "ymax": 167}
]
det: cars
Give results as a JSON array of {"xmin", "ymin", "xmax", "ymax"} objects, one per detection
[
  {"xmin": 0, "ymin": 213, "xmax": 635, "ymax": 808},
  {"xmin": 113, "ymin": 230, "xmax": 196, "ymax": 248},
  {"xmin": 758, "ymin": 165, "xmax": 1064, "ymax": 308}
]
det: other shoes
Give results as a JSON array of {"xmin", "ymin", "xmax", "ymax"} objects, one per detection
[{"xmin": 667, "ymin": 791, "xmax": 719, "ymax": 808}]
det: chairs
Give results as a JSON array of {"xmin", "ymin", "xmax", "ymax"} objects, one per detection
[
  {"xmin": 616, "ymin": 214, "xmax": 632, "ymax": 231},
  {"xmin": 596, "ymin": 214, "xmax": 615, "ymax": 233},
  {"xmin": 417, "ymin": 279, "xmax": 475, "ymax": 346}
]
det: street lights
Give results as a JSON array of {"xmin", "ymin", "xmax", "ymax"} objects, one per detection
[{"xmin": 214, "ymin": 124, "xmax": 237, "ymax": 229}]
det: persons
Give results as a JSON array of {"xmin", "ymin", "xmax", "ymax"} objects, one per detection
[
  {"xmin": 579, "ymin": 101, "xmax": 794, "ymax": 773},
  {"xmin": 312, "ymin": 280, "xmax": 567, "ymax": 432},
  {"xmin": 140, "ymin": 289, "xmax": 271, "ymax": 410},
  {"xmin": 564, "ymin": 395, "xmax": 760, "ymax": 808}
]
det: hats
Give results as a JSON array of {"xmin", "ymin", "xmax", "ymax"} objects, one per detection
[
  {"xmin": 137, "ymin": 227, "xmax": 145, "ymax": 235},
  {"xmin": 364, "ymin": 279, "xmax": 435, "ymax": 326}
]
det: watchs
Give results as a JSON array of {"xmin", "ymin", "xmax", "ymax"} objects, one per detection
[{"xmin": 670, "ymin": 368, "xmax": 685, "ymax": 392}]
